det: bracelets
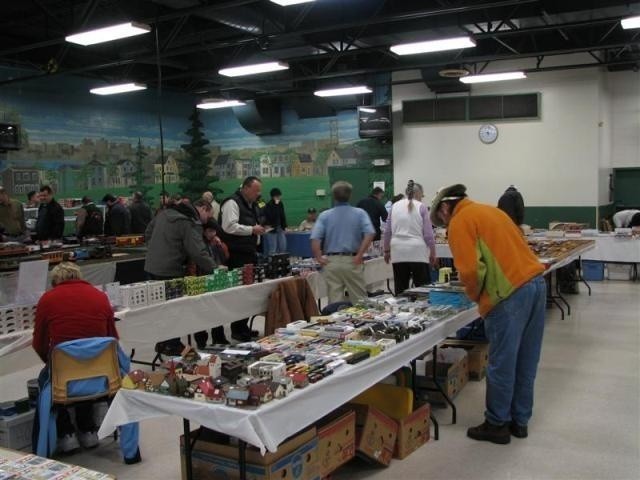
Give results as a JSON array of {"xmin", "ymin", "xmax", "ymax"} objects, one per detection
[{"xmin": 384, "ymin": 249, "xmax": 390, "ymax": 253}]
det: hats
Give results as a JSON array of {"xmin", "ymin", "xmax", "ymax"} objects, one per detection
[{"xmin": 429, "ymin": 183, "xmax": 468, "ymax": 230}]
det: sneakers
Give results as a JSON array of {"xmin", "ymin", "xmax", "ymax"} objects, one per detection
[
  {"xmin": 154, "ymin": 343, "xmax": 187, "ymax": 357},
  {"xmin": 230, "ymin": 326, "xmax": 260, "ymax": 342},
  {"xmin": 77, "ymin": 431, "xmax": 99, "ymax": 449},
  {"xmin": 506, "ymin": 421, "xmax": 529, "ymax": 438},
  {"xmin": 197, "ymin": 337, "xmax": 231, "ymax": 350},
  {"xmin": 55, "ymin": 431, "xmax": 80, "ymax": 458}
]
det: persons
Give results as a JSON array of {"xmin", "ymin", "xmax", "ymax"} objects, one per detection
[
  {"xmin": 27, "ymin": 190, "xmax": 40, "ymax": 202},
  {"xmin": 609, "ymin": 209, "xmax": 640, "ymax": 229},
  {"xmin": 34, "ymin": 185, "xmax": 65, "ymax": 241},
  {"xmin": 379, "ymin": 193, "xmax": 404, "ymax": 236},
  {"xmin": 76, "ymin": 196, "xmax": 102, "ymax": 236},
  {"xmin": 217, "ymin": 176, "xmax": 267, "ymax": 271},
  {"xmin": 186, "ymin": 216, "xmax": 230, "ymax": 277},
  {"xmin": 158, "ymin": 191, "xmax": 169, "ymax": 206},
  {"xmin": 102, "ymin": 194, "xmax": 130, "ymax": 235},
  {"xmin": 383, "ymin": 180, "xmax": 438, "ymax": 296},
  {"xmin": 497, "ymin": 185, "xmax": 524, "ymax": 225},
  {"xmin": 170, "ymin": 192, "xmax": 183, "ymax": 205},
  {"xmin": 262, "ymin": 188, "xmax": 289, "ymax": 260},
  {"xmin": 202, "ymin": 191, "xmax": 221, "ymax": 221},
  {"xmin": 0, "ymin": 187, "xmax": 25, "ymax": 238},
  {"xmin": 299, "ymin": 207, "xmax": 317, "ymax": 231},
  {"xmin": 127, "ymin": 191, "xmax": 153, "ymax": 234},
  {"xmin": 32, "ymin": 260, "xmax": 119, "ymax": 456},
  {"xmin": 309, "ymin": 180, "xmax": 375, "ymax": 306},
  {"xmin": 356, "ymin": 186, "xmax": 388, "ymax": 242},
  {"xmin": 141, "ymin": 198, "xmax": 228, "ymax": 279},
  {"xmin": 430, "ymin": 184, "xmax": 548, "ymax": 445}
]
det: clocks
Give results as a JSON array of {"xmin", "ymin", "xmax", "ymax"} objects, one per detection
[{"xmin": 479, "ymin": 122, "xmax": 499, "ymax": 144}]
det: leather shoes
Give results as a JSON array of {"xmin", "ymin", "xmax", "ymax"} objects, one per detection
[{"xmin": 466, "ymin": 420, "xmax": 511, "ymax": 445}]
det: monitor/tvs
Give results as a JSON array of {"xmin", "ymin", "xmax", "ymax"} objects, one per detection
[
  {"xmin": 357, "ymin": 104, "xmax": 393, "ymax": 138},
  {"xmin": 0, "ymin": 123, "xmax": 22, "ymax": 153}
]
